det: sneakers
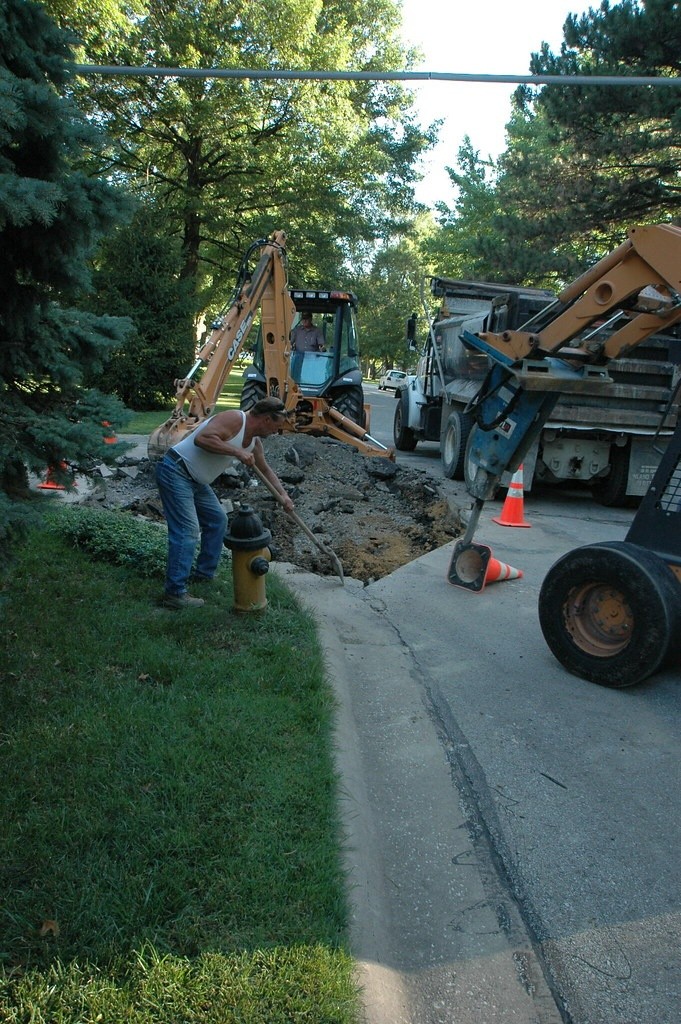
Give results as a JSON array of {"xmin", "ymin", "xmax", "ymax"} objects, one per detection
[{"xmin": 162, "ymin": 590, "xmax": 204, "ymax": 609}]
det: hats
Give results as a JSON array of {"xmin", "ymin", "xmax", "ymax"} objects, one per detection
[
  {"xmin": 300, "ymin": 309, "xmax": 312, "ymax": 321},
  {"xmin": 253, "ymin": 396, "xmax": 286, "ymax": 414}
]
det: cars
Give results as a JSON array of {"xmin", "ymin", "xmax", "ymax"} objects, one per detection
[{"xmin": 379, "ymin": 369, "xmax": 408, "ymax": 390}]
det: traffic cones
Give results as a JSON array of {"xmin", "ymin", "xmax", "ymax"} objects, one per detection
[
  {"xmin": 37, "ymin": 459, "xmax": 77, "ymax": 489},
  {"xmin": 446, "ymin": 538, "xmax": 524, "ymax": 594},
  {"xmin": 492, "ymin": 461, "xmax": 532, "ymax": 528},
  {"xmin": 103, "ymin": 419, "xmax": 117, "ymax": 444}
]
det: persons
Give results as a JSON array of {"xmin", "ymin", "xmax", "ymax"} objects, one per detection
[
  {"xmin": 290, "ymin": 311, "xmax": 326, "ymax": 352},
  {"xmin": 155, "ymin": 397, "xmax": 295, "ymax": 612}
]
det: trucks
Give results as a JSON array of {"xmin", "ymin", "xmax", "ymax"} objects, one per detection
[{"xmin": 394, "ymin": 272, "xmax": 681, "ymax": 508}]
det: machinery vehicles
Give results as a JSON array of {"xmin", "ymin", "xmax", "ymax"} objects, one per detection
[
  {"xmin": 146, "ymin": 228, "xmax": 398, "ymax": 466},
  {"xmin": 460, "ymin": 220, "xmax": 681, "ymax": 689}
]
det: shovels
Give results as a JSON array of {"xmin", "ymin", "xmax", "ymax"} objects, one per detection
[{"xmin": 251, "ymin": 462, "xmax": 344, "ymax": 579}]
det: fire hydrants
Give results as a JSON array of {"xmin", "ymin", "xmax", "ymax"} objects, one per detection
[{"xmin": 224, "ymin": 503, "xmax": 282, "ymax": 614}]
